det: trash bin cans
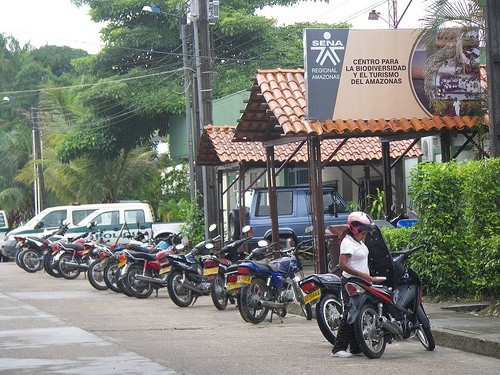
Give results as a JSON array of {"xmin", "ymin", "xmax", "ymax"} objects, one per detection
[
  {"xmin": 325, "ymin": 224, "xmax": 346, "ymax": 275},
  {"xmin": 373, "ymin": 219, "xmax": 395, "ymax": 228},
  {"xmin": 397, "ymin": 219, "xmax": 417, "ymax": 227}
]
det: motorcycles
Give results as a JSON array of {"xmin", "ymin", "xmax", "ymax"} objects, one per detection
[
  {"xmin": 12, "ymin": 219, "xmax": 278, "ymax": 311},
  {"xmin": 340, "ymin": 231, "xmax": 437, "ymax": 360},
  {"xmin": 237, "ymin": 226, "xmax": 313, "ymax": 324},
  {"xmin": 297, "ymin": 262, "xmax": 344, "ymax": 344}
]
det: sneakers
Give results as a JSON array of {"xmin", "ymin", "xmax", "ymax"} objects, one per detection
[{"xmin": 331, "ymin": 350, "xmax": 353, "ymax": 357}]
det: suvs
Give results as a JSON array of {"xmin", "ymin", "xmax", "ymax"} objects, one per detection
[{"xmin": 227, "ymin": 184, "xmax": 356, "ymax": 257}]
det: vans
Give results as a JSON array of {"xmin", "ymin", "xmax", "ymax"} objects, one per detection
[
  {"xmin": 0, "ymin": 199, "xmax": 155, "ymax": 258},
  {"xmin": 1, "ymin": 210, "xmax": 11, "ymax": 264}
]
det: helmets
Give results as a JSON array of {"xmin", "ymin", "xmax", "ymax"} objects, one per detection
[{"xmin": 347, "ymin": 211, "xmax": 375, "ymax": 234}]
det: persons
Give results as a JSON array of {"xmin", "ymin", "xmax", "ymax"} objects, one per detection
[
  {"xmin": 446, "ymin": 96, "xmax": 462, "ymax": 116},
  {"xmin": 330, "ymin": 211, "xmax": 376, "ymax": 357},
  {"xmin": 8, "ymin": 210, "xmax": 24, "ymax": 228}
]
